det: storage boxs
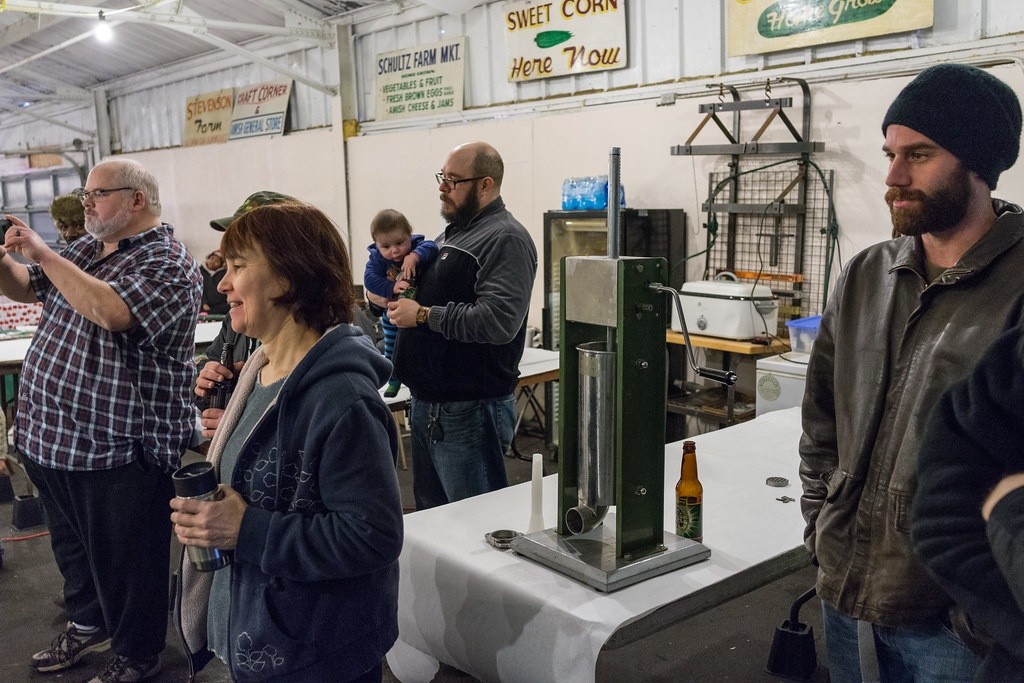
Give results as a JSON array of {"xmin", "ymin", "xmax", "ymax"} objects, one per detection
[{"xmin": 785, "ymin": 316, "xmax": 822, "ymax": 355}]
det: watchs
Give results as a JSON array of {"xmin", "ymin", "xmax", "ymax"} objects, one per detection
[{"xmin": 416, "ymin": 306, "xmax": 430, "ymax": 330}]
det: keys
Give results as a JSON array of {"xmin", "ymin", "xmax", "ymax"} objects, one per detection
[{"xmin": 426, "ymin": 416, "xmax": 444, "ymax": 444}]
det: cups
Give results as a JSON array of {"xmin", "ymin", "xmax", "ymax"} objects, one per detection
[{"xmin": 172, "ymin": 462, "xmax": 232, "ymax": 572}]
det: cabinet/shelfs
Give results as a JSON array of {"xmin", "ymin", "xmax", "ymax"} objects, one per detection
[{"xmin": 667, "ymin": 329, "xmax": 791, "ymax": 428}]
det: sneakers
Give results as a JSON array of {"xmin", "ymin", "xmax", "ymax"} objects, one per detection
[
  {"xmin": 88, "ymin": 650, "xmax": 164, "ymax": 683},
  {"xmin": 29, "ymin": 619, "xmax": 114, "ymax": 673}
]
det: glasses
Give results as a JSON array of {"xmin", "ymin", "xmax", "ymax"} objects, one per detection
[
  {"xmin": 77, "ymin": 187, "xmax": 131, "ymax": 203},
  {"xmin": 435, "ymin": 172, "xmax": 488, "ymax": 191}
]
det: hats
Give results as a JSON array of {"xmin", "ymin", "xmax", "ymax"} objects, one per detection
[
  {"xmin": 210, "ymin": 192, "xmax": 304, "ymax": 231},
  {"xmin": 882, "ymin": 63, "xmax": 1023, "ymax": 191},
  {"xmin": 51, "ymin": 189, "xmax": 85, "ymax": 235}
]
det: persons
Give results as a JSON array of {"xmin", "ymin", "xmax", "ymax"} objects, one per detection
[
  {"xmin": 363, "ymin": 209, "xmax": 439, "ymax": 398},
  {"xmin": 0, "ymin": 160, "xmax": 300, "ymax": 683},
  {"xmin": 798, "ymin": 64, "xmax": 1024, "ymax": 683},
  {"xmin": 365, "ymin": 141, "xmax": 538, "ymax": 513},
  {"xmin": 169, "ymin": 204, "xmax": 403, "ymax": 683}
]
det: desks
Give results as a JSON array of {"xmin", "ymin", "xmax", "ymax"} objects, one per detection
[
  {"xmin": 187, "ymin": 346, "xmax": 561, "ymax": 511},
  {"xmin": 401, "ymin": 408, "xmax": 822, "ymax": 679},
  {"xmin": 0, "ymin": 321, "xmax": 222, "ymax": 431}
]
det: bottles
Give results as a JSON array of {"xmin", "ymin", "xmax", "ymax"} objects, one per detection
[
  {"xmin": 675, "ymin": 441, "xmax": 704, "ymax": 543},
  {"xmin": 398, "ymin": 262, "xmax": 420, "ymax": 300},
  {"xmin": 561, "ymin": 176, "xmax": 625, "ymax": 208},
  {"xmin": 207, "ymin": 343, "xmax": 235, "ymax": 432}
]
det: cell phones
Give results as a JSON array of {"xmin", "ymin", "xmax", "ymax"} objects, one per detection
[{"xmin": 0, "ymin": 219, "xmax": 12, "ymax": 246}]
